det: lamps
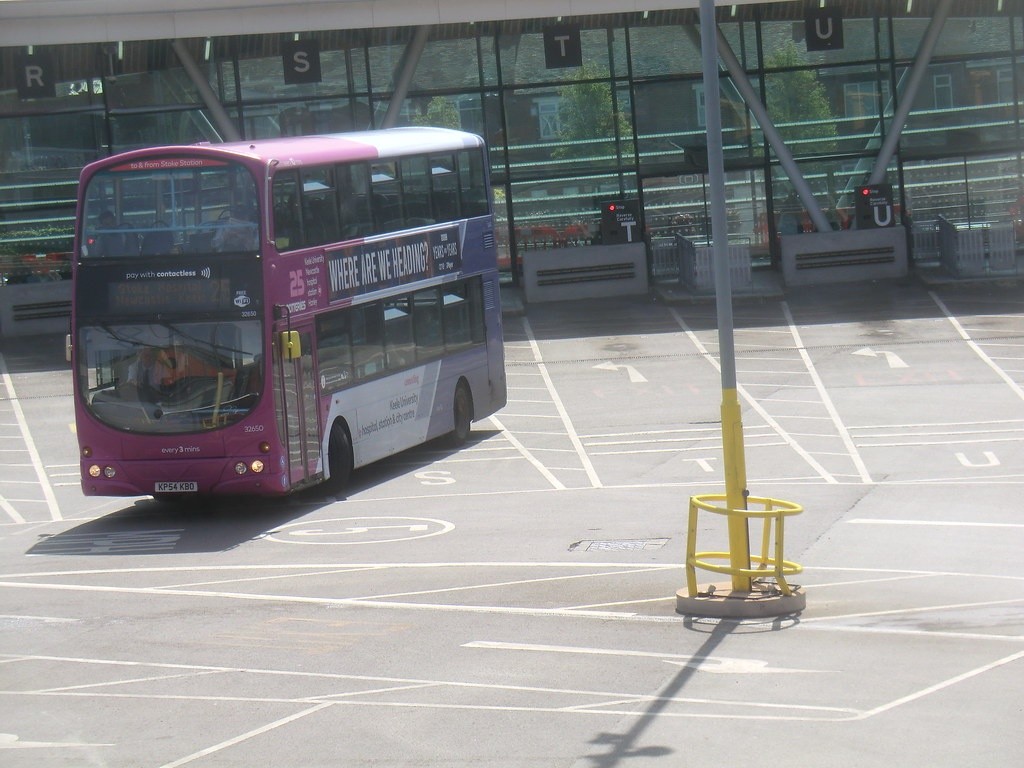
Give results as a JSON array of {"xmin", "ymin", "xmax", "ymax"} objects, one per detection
[
  {"xmin": 995, "ymin": 0, "xmax": 1004, "ymax": 15},
  {"xmin": 204, "ymin": 35, "xmax": 212, "ymax": 60},
  {"xmin": 818, "ymin": 0, "xmax": 826, "ymax": 8},
  {"xmin": 904, "ymin": 0, "xmax": 913, "ymax": 15},
  {"xmin": 118, "ymin": 42, "xmax": 126, "ymax": 65},
  {"xmin": 641, "ymin": 10, "xmax": 650, "ymax": 22},
  {"xmin": 27, "ymin": 47, "xmax": 36, "ymax": 60},
  {"xmin": 730, "ymin": 5, "xmax": 737, "ymax": 18}
]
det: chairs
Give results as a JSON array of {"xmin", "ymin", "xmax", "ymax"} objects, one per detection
[{"xmin": 117, "ymin": 188, "xmax": 480, "ymax": 257}]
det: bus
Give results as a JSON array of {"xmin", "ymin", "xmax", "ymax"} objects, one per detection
[{"xmin": 64, "ymin": 125, "xmax": 508, "ymax": 506}]
